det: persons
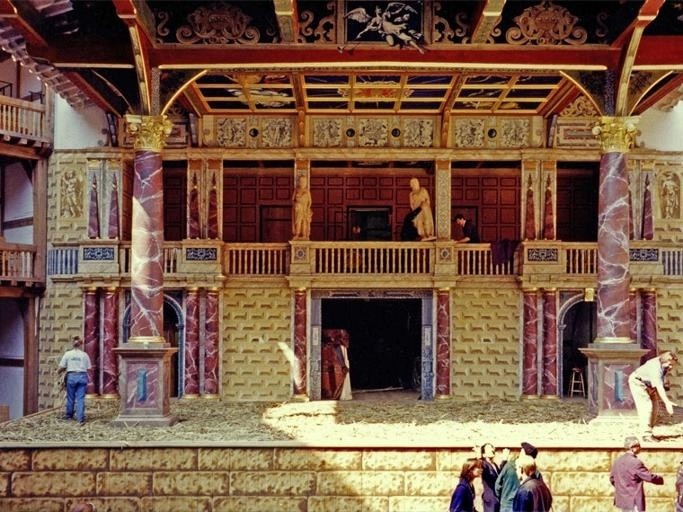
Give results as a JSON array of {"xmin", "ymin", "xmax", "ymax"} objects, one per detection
[
  {"xmin": 409, "ymin": 177, "xmax": 435, "ymax": 239},
  {"xmin": 610, "ymin": 437, "xmax": 664, "ymax": 512},
  {"xmin": 450, "ymin": 441, "xmax": 551, "ymax": 512},
  {"xmin": 628, "ymin": 351, "xmax": 678, "ymax": 442},
  {"xmin": 452, "ymin": 214, "xmax": 481, "ymax": 274},
  {"xmin": 401, "ymin": 201, "xmax": 425, "ymax": 241},
  {"xmin": 674, "ymin": 461, "xmax": 683, "ymax": 512},
  {"xmin": 57, "ymin": 339, "xmax": 92, "ymax": 426}
]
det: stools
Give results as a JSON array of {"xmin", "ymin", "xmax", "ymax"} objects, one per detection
[{"xmin": 567, "ymin": 367, "xmax": 586, "ymax": 398}]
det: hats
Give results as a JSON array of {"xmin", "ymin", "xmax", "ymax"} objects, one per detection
[{"xmin": 521, "ymin": 441, "xmax": 537, "ymax": 458}]
